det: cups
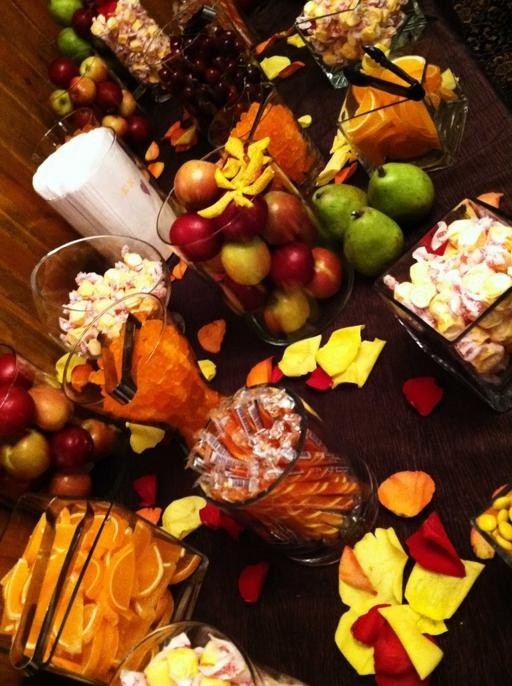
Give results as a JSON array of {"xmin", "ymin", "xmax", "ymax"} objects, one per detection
[
  {"xmin": 29, "ymin": 0, "xmax": 325, "ymax": 278},
  {"xmin": 0, "ymin": 492, "xmax": 209, "ymax": 685},
  {"xmin": 292, "ymin": 1, "xmax": 469, "ymax": 173},
  {"xmin": 155, "ymin": 138, "xmax": 355, "ymax": 346},
  {"xmin": 470, "ymin": 480, "xmax": 512, "ymax": 568},
  {"xmin": 108, "ymin": 620, "xmax": 305, "ymax": 685},
  {"xmin": 194, "ymin": 382, "xmax": 379, "ymax": 566},
  {"xmin": 0, "ymin": 235, "xmax": 225, "ymax": 501},
  {"xmin": 372, "ymin": 198, "xmax": 512, "ymax": 412}
]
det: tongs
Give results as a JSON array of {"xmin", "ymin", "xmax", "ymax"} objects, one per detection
[{"xmin": 9, "ymin": 496, "xmax": 95, "ymax": 677}]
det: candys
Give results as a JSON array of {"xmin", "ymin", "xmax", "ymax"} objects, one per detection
[
  {"xmin": 0, "ymin": 246, "xmax": 369, "ymax": 686},
  {"xmin": 90, "ymin": 0, "xmax": 442, "ymax": 184},
  {"xmin": 383, "ymin": 216, "xmax": 512, "ymax": 374}
]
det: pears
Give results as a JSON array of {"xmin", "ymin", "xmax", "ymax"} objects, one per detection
[{"xmin": 311, "ymin": 161, "xmax": 436, "ymax": 283}]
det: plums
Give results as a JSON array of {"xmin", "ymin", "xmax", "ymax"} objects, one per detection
[
  {"xmin": 48, "ymin": 0, "xmax": 149, "ymax": 144},
  {"xmin": 169, "ymin": 158, "xmax": 342, "ymax": 333}
]
